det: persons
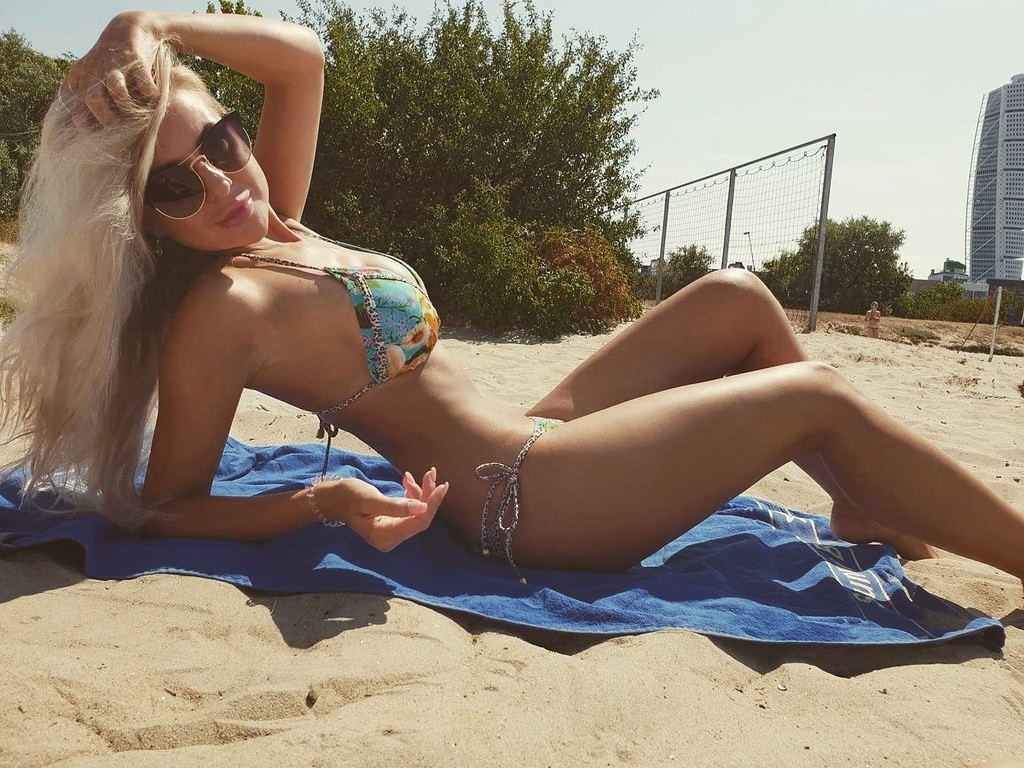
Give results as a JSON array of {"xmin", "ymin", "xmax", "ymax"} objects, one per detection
[
  {"xmin": 864, "ymin": 301, "xmax": 881, "ymax": 339},
  {"xmin": 2, "ymin": 1, "xmax": 1023, "ymax": 584}
]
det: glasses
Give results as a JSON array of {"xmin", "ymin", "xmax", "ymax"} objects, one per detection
[{"xmin": 144, "ymin": 109, "xmax": 252, "ymax": 219}]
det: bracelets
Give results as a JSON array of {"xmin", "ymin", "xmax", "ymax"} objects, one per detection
[{"xmin": 305, "ymin": 475, "xmax": 346, "ymax": 527}]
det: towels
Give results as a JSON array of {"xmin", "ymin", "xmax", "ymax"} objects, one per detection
[{"xmin": 1, "ymin": 415, "xmax": 1012, "ymax": 658}]
